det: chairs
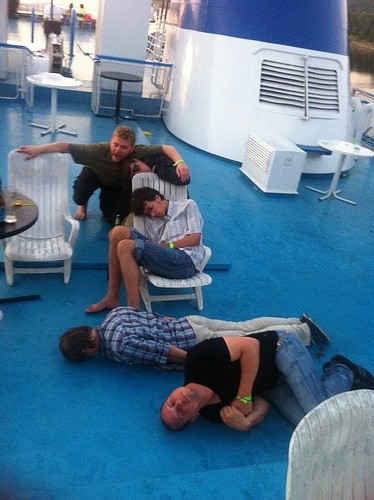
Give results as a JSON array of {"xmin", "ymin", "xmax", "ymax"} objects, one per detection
[
  {"xmin": 131, "ymin": 170, "xmax": 213, "ymax": 314},
  {"xmin": 3, "ymin": 144, "xmax": 81, "ymax": 286},
  {"xmin": 281, "ymin": 388, "xmax": 374, "ymax": 500}
]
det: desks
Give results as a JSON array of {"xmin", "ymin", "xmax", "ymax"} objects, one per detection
[
  {"xmin": 25, "ymin": 71, "xmax": 85, "ymax": 139},
  {"xmin": 94, "ymin": 68, "xmax": 143, "ymax": 124},
  {"xmin": 306, "ymin": 139, "xmax": 374, "ymax": 205},
  {"xmin": 0, "ymin": 187, "xmax": 41, "ymax": 304}
]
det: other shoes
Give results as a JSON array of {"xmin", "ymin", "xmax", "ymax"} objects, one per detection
[
  {"xmin": 300, "ymin": 314, "xmax": 328, "ymax": 342},
  {"xmin": 331, "ymin": 354, "xmax": 372, "ymax": 381},
  {"xmin": 323, "ymin": 362, "xmax": 364, "ymax": 388}
]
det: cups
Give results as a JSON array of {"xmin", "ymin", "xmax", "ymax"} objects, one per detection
[{"xmin": 4, "ymin": 207, "xmax": 18, "ymax": 223}]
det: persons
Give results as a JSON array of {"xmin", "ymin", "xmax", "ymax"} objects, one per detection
[
  {"xmin": 160, "ymin": 330, "xmax": 374, "ymax": 431},
  {"xmin": 65, "ymin": 3, "xmax": 73, "ymax": 26},
  {"xmin": 84, "ymin": 187, "xmax": 204, "ymax": 312},
  {"xmin": 59, "ymin": 307, "xmax": 329, "ymax": 371},
  {"xmin": 16, "ymin": 125, "xmax": 192, "ymax": 225},
  {"xmin": 77, "ymin": 4, "xmax": 85, "ymax": 28}
]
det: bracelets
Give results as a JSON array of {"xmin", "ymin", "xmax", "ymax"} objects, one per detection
[
  {"xmin": 235, "ymin": 396, "xmax": 251, "ymax": 403},
  {"xmin": 173, "ymin": 160, "xmax": 184, "ymax": 167},
  {"xmin": 168, "ymin": 241, "xmax": 174, "ymax": 249}
]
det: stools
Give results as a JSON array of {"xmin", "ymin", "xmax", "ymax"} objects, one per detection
[{"xmin": 298, "ymin": 145, "xmax": 332, "ymax": 156}]
